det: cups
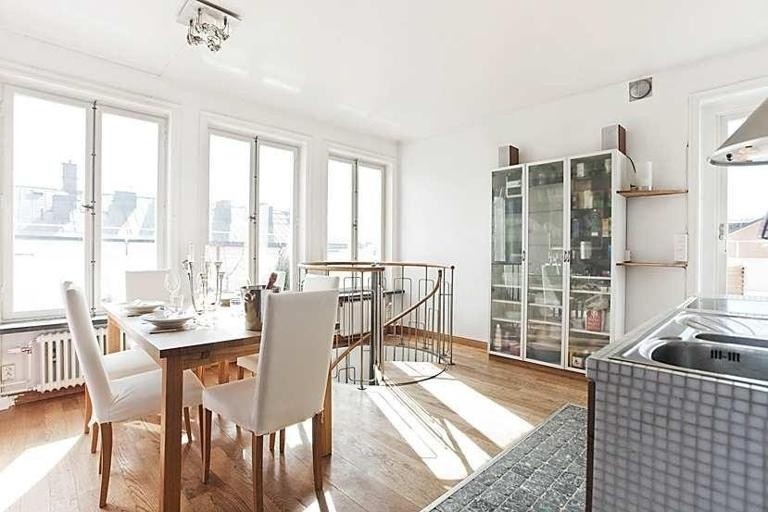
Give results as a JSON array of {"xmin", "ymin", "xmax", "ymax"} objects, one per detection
[{"xmin": 580, "ymin": 241, "xmax": 593, "ymax": 261}]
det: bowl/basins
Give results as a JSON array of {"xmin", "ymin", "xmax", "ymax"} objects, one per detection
[
  {"xmin": 140, "ymin": 312, "xmax": 194, "ymax": 328},
  {"xmin": 120, "ymin": 303, "xmax": 162, "ymax": 315}
]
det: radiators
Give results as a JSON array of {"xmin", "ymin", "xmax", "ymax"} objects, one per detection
[{"xmin": 32, "ymin": 326, "xmax": 126, "ymax": 395}]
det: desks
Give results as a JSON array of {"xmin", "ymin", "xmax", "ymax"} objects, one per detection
[{"xmin": 99, "ymin": 294, "xmax": 337, "ymax": 512}]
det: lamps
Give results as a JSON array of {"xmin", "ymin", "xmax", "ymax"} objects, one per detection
[{"xmin": 175, "ymin": 0, "xmax": 241, "ymax": 53}]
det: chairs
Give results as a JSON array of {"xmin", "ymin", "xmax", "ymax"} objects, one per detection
[
  {"xmin": 60, "ymin": 280, "xmax": 207, "ymax": 509},
  {"xmin": 194, "ymin": 287, "xmax": 338, "ymax": 509},
  {"xmin": 231, "ymin": 271, "xmax": 338, "ymax": 463},
  {"xmin": 123, "ymin": 270, "xmax": 219, "ymax": 446}
]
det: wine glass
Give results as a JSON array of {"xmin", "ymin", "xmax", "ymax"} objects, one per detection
[{"xmin": 164, "ymin": 271, "xmax": 180, "ymax": 313}]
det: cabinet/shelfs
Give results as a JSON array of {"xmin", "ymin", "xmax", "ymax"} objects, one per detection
[{"xmin": 489, "ymin": 150, "xmax": 687, "ymax": 375}]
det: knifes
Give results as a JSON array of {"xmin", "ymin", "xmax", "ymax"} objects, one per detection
[{"xmin": 150, "ymin": 327, "xmax": 190, "ymax": 334}]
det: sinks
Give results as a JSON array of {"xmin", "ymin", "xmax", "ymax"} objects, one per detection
[
  {"xmin": 694, "ymin": 329, "xmax": 767, "ymax": 347},
  {"xmin": 640, "ymin": 334, "xmax": 767, "ymax": 384}
]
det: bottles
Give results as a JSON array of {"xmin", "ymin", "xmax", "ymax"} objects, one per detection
[
  {"xmin": 590, "ymin": 209, "xmax": 601, "ymax": 237},
  {"xmin": 494, "ymin": 323, "xmax": 510, "ymax": 351},
  {"xmin": 264, "ymin": 272, "xmax": 277, "ymax": 290},
  {"xmin": 572, "ymin": 218, "xmax": 584, "ymax": 241}
]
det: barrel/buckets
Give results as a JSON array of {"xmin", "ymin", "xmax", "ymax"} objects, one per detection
[{"xmin": 241, "ymin": 285, "xmax": 280, "ymax": 331}]
